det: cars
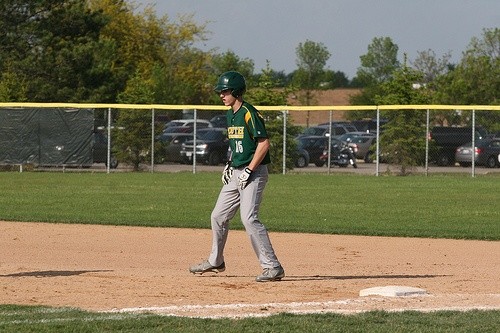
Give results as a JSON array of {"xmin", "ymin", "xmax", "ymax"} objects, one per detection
[
  {"xmin": 93, "ymin": 128, "xmax": 119, "ymax": 169},
  {"xmin": 292, "ymin": 120, "xmax": 387, "ymax": 169},
  {"xmin": 155, "ymin": 114, "xmax": 228, "ymax": 166},
  {"xmin": 455, "ymin": 136, "xmax": 500, "ymax": 168}
]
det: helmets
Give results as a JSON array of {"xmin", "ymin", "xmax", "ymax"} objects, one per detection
[{"xmin": 213, "ymin": 71, "xmax": 247, "ymax": 100}]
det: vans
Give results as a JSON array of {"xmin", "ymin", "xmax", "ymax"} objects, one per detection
[{"xmin": 429, "ymin": 126, "xmax": 473, "ymax": 166}]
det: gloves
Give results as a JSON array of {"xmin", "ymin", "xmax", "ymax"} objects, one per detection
[
  {"xmin": 238, "ymin": 167, "xmax": 253, "ymax": 191},
  {"xmin": 221, "ymin": 161, "xmax": 233, "ymax": 185}
]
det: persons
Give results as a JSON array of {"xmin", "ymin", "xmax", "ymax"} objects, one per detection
[{"xmin": 189, "ymin": 71, "xmax": 285, "ymax": 283}]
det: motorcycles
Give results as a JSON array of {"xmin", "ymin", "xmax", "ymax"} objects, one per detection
[{"xmin": 319, "ymin": 137, "xmax": 358, "ymax": 169}]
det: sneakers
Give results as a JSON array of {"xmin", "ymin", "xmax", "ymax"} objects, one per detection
[
  {"xmin": 255, "ymin": 263, "xmax": 285, "ymax": 282},
  {"xmin": 189, "ymin": 258, "xmax": 226, "ymax": 274}
]
